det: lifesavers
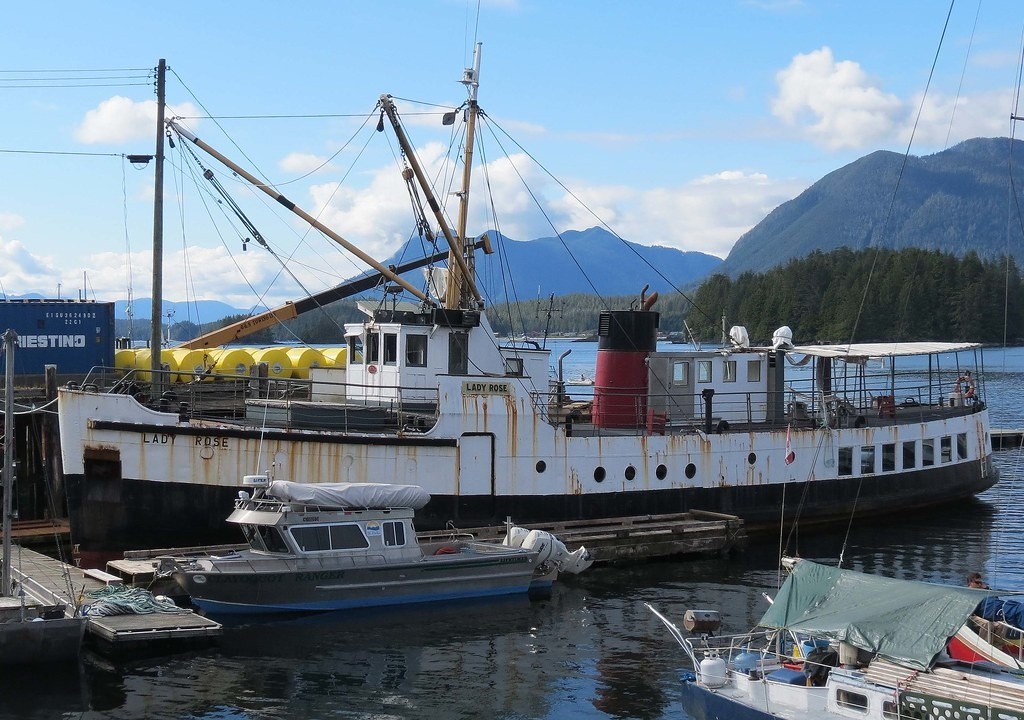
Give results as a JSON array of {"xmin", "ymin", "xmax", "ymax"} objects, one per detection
[
  {"xmin": 435, "ymin": 545, "xmax": 457, "ymax": 554},
  {"xmin": 954, "ymin": 376, "xmax": 975, "ymax": 397}
]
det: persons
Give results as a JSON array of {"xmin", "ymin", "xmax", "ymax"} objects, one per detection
[{"xmin": 966, "ymin": 571, "xmax": 992, "ymax": 619}]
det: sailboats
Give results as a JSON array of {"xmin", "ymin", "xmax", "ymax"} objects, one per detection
[{"xmin": 644, "ymin": 0, "xmax": 1022, "ymax": 720}]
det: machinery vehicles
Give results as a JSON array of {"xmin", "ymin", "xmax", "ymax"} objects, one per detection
[{"xmin": 166, "ymin": 235, "xmax": 495, "ymax": 349}]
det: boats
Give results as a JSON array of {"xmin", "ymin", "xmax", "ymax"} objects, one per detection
[
  {"xmin": 169, "ymin": 456, "xmax": 596, "ymax": 614},
  {"xmin": 58, "ymin": 0, "xmax": 999, "ymax": 549}
]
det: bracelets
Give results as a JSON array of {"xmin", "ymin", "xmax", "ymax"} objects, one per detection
[{"xmin": 985, "ymin": 586, "xmax": 986, "ymax": 588}]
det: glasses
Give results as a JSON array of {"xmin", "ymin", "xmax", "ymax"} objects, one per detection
[{"xmin": 973, "ymin": 581, "xmax": 982, "ymax": 584}]
